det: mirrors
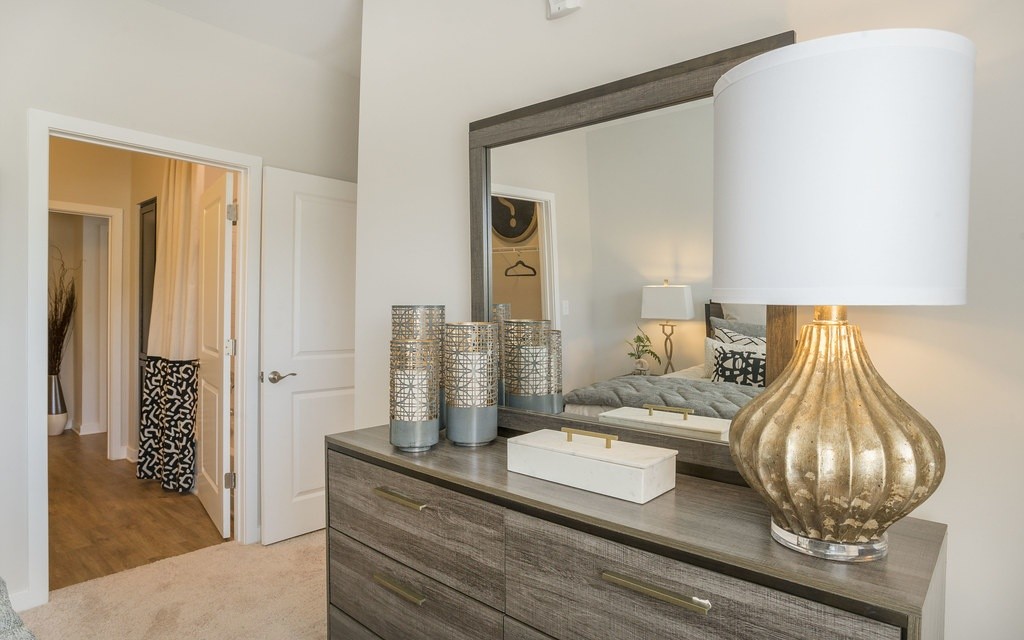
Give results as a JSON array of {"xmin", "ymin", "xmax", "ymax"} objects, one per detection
[{"xmin": 464, "ymin": 28, "xmax": 796, "ymax": 474}]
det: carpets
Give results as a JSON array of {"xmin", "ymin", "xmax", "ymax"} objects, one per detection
[{"xmin": 17, "ymin": 527, "xmax": 330, "ymax": 640}]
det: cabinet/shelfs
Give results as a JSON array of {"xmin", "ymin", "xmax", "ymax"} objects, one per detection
[{"xmin": 321, "ymin": 423, "xmax": 949, "ymax": 640}]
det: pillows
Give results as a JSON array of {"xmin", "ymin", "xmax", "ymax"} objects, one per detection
[
  {"xmin": 709, "ymin": 314, "xmax": 766, "ymax": 338},
  {"xmin": 710, "ymin": 344, "xmax": 765, "ymax": 387},
  {"xmin": 700, "ymin": 336, "xmax": 766, "ymax": 381},
  {"xmin": 714, "ymin": 327, "xmax": 767, "ymax": 346}
]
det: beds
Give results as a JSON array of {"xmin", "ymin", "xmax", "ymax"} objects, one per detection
[{"xmin": 560, "ymin": 298, "xmax": 767, "ymax": 421}]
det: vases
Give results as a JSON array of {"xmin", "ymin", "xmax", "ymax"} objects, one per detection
[{"xmin": 46, "ymin": 374, "xmax": 70, "ymax": 439}]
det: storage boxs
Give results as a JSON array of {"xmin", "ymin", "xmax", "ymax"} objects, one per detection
[
  {"xmin": 503, "ymin": 426, "xmax": 681, "ymax": 507},
  {"xmin": 594, "ymin": 402, "xmax": 734, "ymax": 444}
]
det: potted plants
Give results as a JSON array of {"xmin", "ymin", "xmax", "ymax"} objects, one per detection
[{"xmin": 624, "ymin": 321, "xmax": 662, "ymax": 376}]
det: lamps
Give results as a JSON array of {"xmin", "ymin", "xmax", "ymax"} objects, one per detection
[
  {"xmin": 639, "ymin": 279, "xmax": 696, "ymax": 375},
  {"xmin": 706, "ymin": 24, "xmax": 979, "ymax": 569}
]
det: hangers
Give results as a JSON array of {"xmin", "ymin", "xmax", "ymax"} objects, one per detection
[{"xmin": 503, "ymin": 250, "xmax": 537, "ymax": 278}]
